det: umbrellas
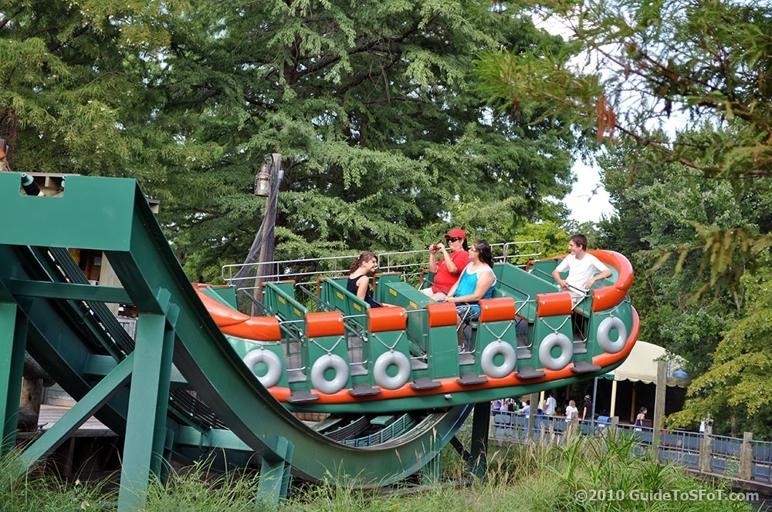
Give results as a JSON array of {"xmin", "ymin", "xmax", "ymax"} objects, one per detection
[{"xmin": 606, "ymin": 340, "xmax": 696, "ymax": 418}]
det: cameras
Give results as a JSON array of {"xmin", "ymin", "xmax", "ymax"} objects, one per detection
[{"xmin": 432, "ymin": 245, "xmax": 440, "ymax": 251}]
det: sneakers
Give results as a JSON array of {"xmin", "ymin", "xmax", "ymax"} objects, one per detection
[{"xmin": 458, "ymin": 343, "xmax": 465, "ymax": 354}]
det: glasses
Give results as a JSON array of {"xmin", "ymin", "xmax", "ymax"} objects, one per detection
[{"xmin": 446, "ymin": 239, "xmax": 460, "ymax": 242}]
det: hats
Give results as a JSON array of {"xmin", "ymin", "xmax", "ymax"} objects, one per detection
[{"xmin": 445, "ymin": 229, "xmax": 465, "ymax": 238}]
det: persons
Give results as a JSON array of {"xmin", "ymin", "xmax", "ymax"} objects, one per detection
[
  {"xmin": 418, "ymin": 226, "xmax": 471, "ymax": 302},
  {"xmin": 552, "ymin": 234, "xmax": 613, "ymax": 308},
  {"xmin": 634, "ymin": 407, "xmax": 648, "ymax": 431},
  {"xmin": 436, "ymin": 239, "xmax": 497, "ymax": 354},
  {"xmin": 347, "ymin": 252, "xmax": 409, "ymax": 322},
  {"xmin": 490, "ymin": 392, "xmax": 592, "ymax": 424}
]
